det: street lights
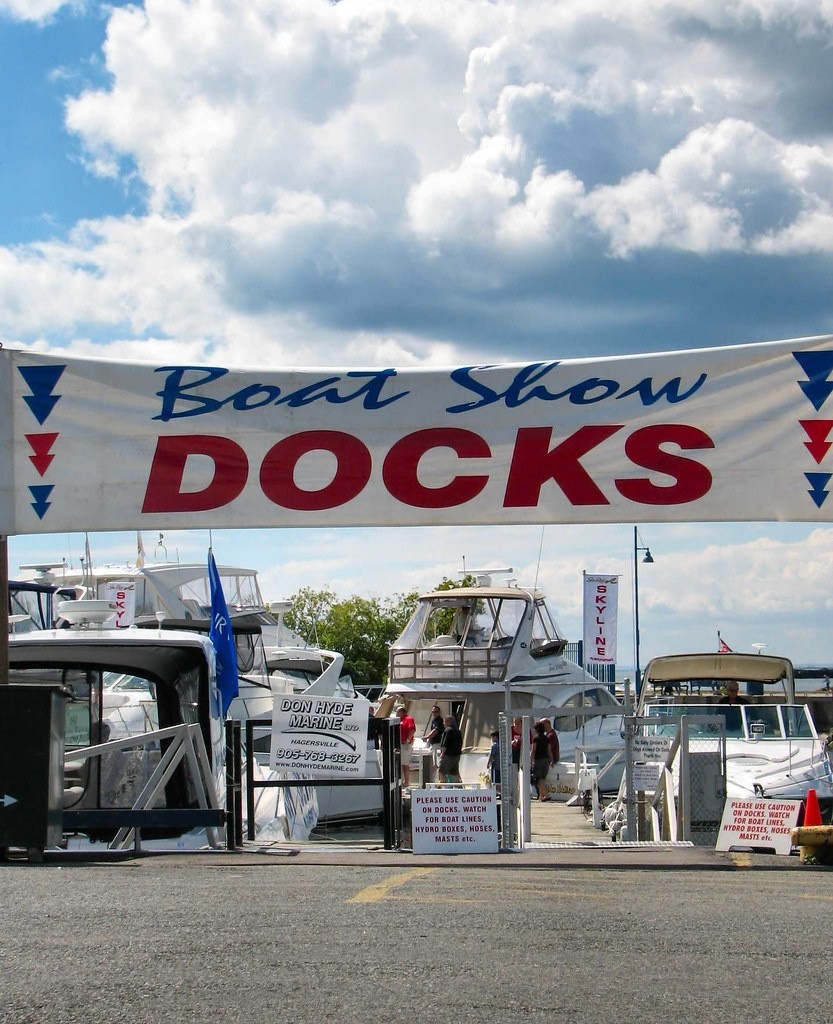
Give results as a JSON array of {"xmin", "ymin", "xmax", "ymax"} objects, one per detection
[{"xmin": 629, "ymin": 522, "xmax": 655, "ymax": 694}]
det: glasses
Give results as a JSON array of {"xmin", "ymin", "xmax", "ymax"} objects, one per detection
[
  {"xmin": 728, "ymin": 688, "xmax": 738, "ymax": 692},
  {"xmin": 493, "ymin": 735, "xmax": 495, "ymax": 737},
  {"xmin": 432, "ymin": 711, "xmax": 439, "ymax": 713}
]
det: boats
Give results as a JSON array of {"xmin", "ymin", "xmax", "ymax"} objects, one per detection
[{"xmin": 8, "ymin": 528, "xmax": 833, "ymax": 852}]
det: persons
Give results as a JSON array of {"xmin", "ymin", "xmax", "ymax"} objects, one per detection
[
  {"xmin": 714, "ymin": 681, "xmax": 761, "ymax": 731},
  {"xmin": 510, "ymin": 716, "xmax": 560, "ymax": 802},
  {"xmin": 368, "ymin": 707, "xmax": 375, "ymax": 718},
  {"xmin": 421, "ymin": 705, "xmax": 446, "ymax": 782},
  {"xmin": 486, "ymin": 730, "xmax": 501, "ymax": 800},
  {"xmin": 394, "ymin": 706, "xmax": 416, "ymax": 787},
  {"xmin": 456, "ymin": 605, "xmax": 483, "ymax": 647},
  {"xmin": 437, "ymin": 715, "xmax": 463, "ymax": 789},
  {"xmin": 77, "ymin": 721, "xmax": 110, "ymax": 801},
  {"xmin": 823, "ymin": 675, "xmax": 830, "ymax": 690}
]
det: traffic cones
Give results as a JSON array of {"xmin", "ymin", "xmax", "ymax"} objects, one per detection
[{"xmin": 799, "ymin": 789, "xmax": 825, "ymax": 863}]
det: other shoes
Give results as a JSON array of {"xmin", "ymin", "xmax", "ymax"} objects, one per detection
[{"xmin": 540, "ymin": 796, "xmax": 551, "ymax": 802}]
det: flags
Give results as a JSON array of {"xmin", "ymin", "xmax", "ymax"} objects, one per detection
[
  {"xmin": 135, "ymin": 532, "xmax": 146, "ymax": 571},
  {"xmin": 720, "ymin": 638, "xmax": 733, "ymax": 652},
  {"xmin": 204, "ymin": 549, "xmax": 239, "ymax": 719}
]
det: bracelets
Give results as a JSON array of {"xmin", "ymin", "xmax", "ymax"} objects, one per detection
[{"xmin": 406, "ymin": 739, "xmax": 410, "ymax": 742}]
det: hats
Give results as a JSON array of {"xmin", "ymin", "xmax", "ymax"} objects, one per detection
[
  {"xmin": 491, "ymin": 731, "xmax": 499, "ymax": 739},
  {"xmin": 534, "ymin": 723, "xmax": 545, "ymax": 732}
]
